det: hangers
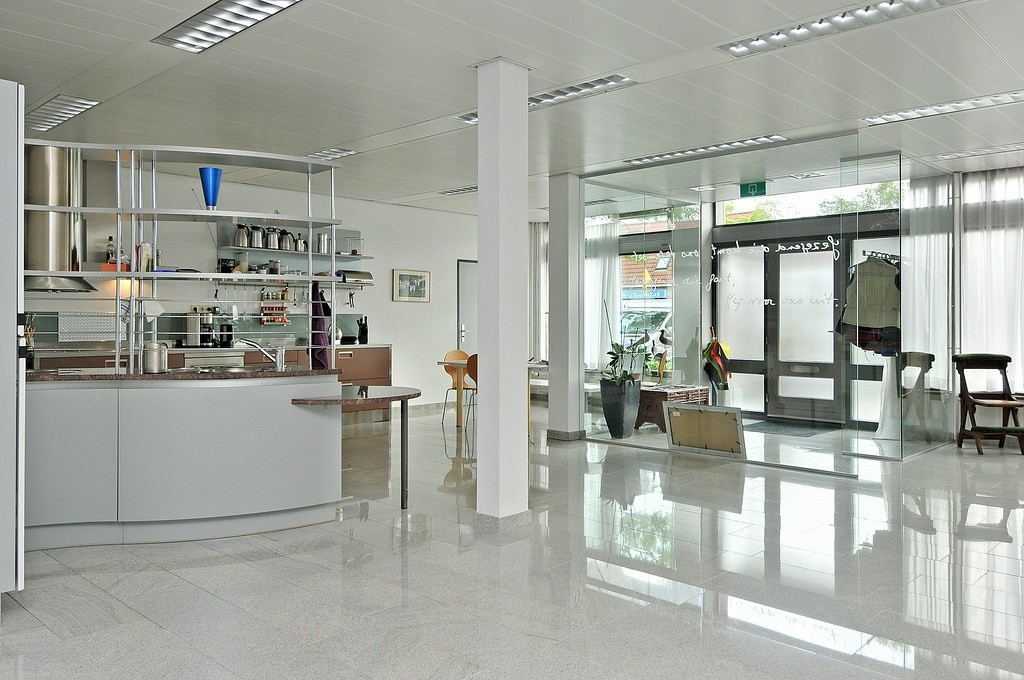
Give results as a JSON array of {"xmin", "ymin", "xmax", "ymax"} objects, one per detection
[{"xmin": 848, "ymin": 251, "xmax": 899, "ymax": 275}]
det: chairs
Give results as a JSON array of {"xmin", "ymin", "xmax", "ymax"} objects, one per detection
[
  {"xmin": 442, "ymin": 350, "xmax": 476, "ymax": 425},
  {"xmin": 901, "ymin": 352, "xmax": 935, "ymax": 444},
  {"xmin": 952, "ymin": 354, "xmax": 1024, "ymax": 455},
  {"xmin": 463, "ymin": 354, "xmax": 478, "ymax": 428},
  {"xmin": 465, "ymin": 428, "xmax": 477, "ymax": 509},
  {"xmin": 442, "ymin": 425, "xmax": 473, "ymax": 485}
]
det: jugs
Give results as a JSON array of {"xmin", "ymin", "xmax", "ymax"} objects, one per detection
[
  {"xmin": 234, "ymin": 223, "xmax": 249, "ymax": 248},
  {"xmin": 265, "ymin": 227, "xmax": 281, "ymax": 250},
  {"xmin": 277, "ymin": 229, "xmax": 295, "ymax": 251},
  {"xmin": 249, "ymin": 226, "xmax": 265, "ymax": 248},
  {"xmin": 294, "ymin": 239, "xmax": 307, "ymax": 252}
]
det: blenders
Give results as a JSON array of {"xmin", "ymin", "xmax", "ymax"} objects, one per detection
[{"xmin": 182, "ymin": 313, "xmax": 216, "ymax": 346}]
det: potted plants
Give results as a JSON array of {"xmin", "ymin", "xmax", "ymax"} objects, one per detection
[{"xmin": 600, "ymin": 343, "xmax": 652, "ymax": 438}]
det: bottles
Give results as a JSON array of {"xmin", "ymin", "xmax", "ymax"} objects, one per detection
[
  {"xmin": 105, "ymin": 236, "xmax": 115, "ymax": 263},
  {"xmin": 258, "ymin": 288, "xmax": 290, "ymax": 326},
  {"xmin": 249, "ymin": 260, "xmax": 301, "ymax": 275},
  {"xmin": 116, "ymin": 236, "xmax": 123, "ymax": 260}
]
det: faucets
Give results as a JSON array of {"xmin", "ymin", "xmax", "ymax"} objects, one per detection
[{"xmin": 240, "ymin": 338, "xmax": 286, "ymax": 373}]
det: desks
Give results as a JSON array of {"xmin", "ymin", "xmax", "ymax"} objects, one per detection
[
  {"xmin": 437, "ymin": 362, "xmax": 549, "ymax": 437},
  {"xmin": 634, "ymin": 386, "xmax": 709, "ymax": 433}
]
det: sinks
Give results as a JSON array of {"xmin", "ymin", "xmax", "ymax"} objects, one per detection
[{"xmin": 219, "ymin": 367, "xmax": 255, "ymax": 374}]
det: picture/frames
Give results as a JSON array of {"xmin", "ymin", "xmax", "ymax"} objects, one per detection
[{"xmin": 392, "ymin": 269, "xmax": 431, "ymax": 303}]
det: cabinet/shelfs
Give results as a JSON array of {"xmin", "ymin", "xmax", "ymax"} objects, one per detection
[
  {"xmin": 218, "ymin": 222, "xmax": 375, "ymax": 289},
  {"xmin": 342, "ymin": 410, "xmax": 391, "ymax": 500},
  {"xmin": 40, "ymin": 353, "xmax": 184, "ymax": 369},
  {"xmin": 297, "ymin": 347, "xmax": 389, "ymax": 412},
  {"xmin": 245, "ymin": 350, "xmax": 297, "ymax": 367},
  {"xmin": 23, "ymin": 138, "xmax": 421, "ymax": 552}
]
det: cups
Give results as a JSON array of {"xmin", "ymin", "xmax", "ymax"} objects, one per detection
[
  {"xmin": 239, "ymin": 252, "xmax": 250, "ymax": 272},
  {"xmin": 317, "ymin": 232, "xmax": 328, "ymax": 253}
]
